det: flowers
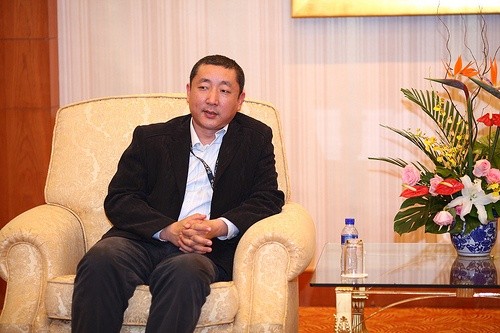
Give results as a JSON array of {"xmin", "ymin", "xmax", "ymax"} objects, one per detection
[{"xmin": 366, "ymin": 3, "xmax": 499, "ymax": 236}]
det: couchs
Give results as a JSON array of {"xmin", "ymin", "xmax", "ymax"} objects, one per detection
[{"xmin": 0, "ymin": 93, "xmax": 315, "ymax": 333}]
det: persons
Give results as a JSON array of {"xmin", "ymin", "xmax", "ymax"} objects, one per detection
[{"xmin": 67, "ymin": 55, "xmax": 290, "ymax": 332}]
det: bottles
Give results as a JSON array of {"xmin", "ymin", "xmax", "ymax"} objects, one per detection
[{"xmin": 341, "ymin": 218, "xmax": 358, "ymax": 274}]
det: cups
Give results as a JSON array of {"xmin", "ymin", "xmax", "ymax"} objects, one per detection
[{"xmin": 344, "ymin": 238, "xmax": 364, "ymax": 275}]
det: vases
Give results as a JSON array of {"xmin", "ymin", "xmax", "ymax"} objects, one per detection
[
  {"xmin": 449, "ymin": 256, "xmax": 497, "ymax": 286},
  {"xmin": 449, "ymin": 212, "xmax": 497, "ymax": 256}
]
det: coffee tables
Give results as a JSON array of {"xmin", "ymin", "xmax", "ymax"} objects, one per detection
[{"xmin": 308, "ymin": 241, "xmax": 500, "ymax": 333}]
